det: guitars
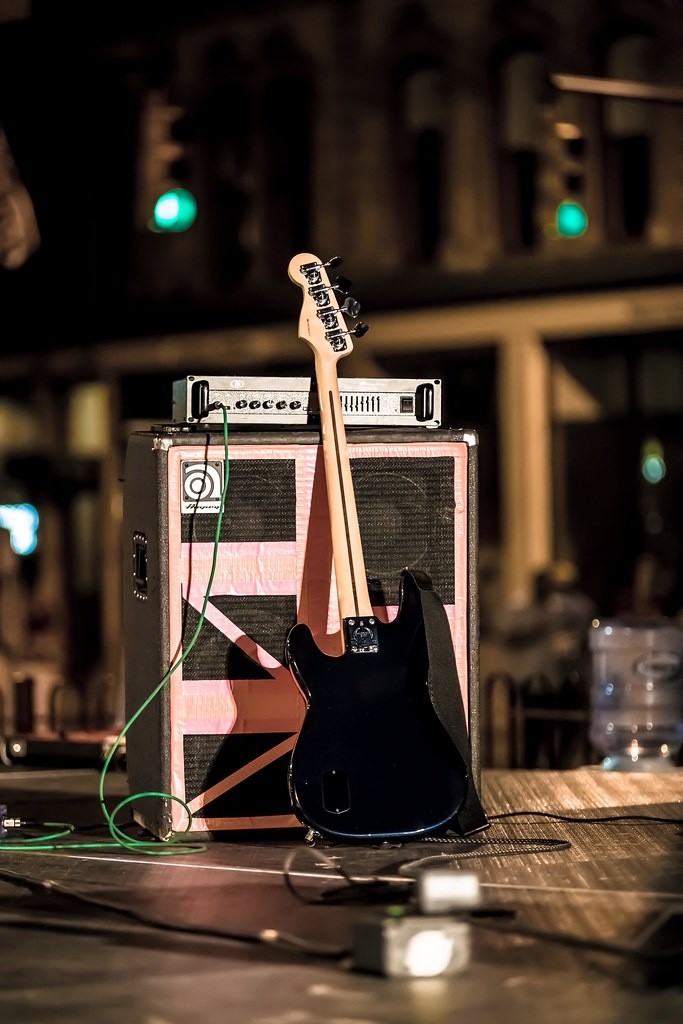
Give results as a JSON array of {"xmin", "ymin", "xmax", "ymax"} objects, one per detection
[{"xmin": 281, "ymin": 248, "xmax": 473, "ymax": 848}]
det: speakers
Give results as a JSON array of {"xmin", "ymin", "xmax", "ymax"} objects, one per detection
[{"xmin": 120, "ymin": 424, "xmax": 479, "ymax": 846}]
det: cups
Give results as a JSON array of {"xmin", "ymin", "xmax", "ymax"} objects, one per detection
[{"xmin": 589, "ymin": 612, "xmax": 683, "ymax": 771}]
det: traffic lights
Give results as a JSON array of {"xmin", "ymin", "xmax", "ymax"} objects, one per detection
[
  {"xmin": 533, "ymin": 123, "xmax": 595, "ymax": 244},
  {"xmin": 134, "ymin": 97, "xmax": 200, "ymax": 238}
]
